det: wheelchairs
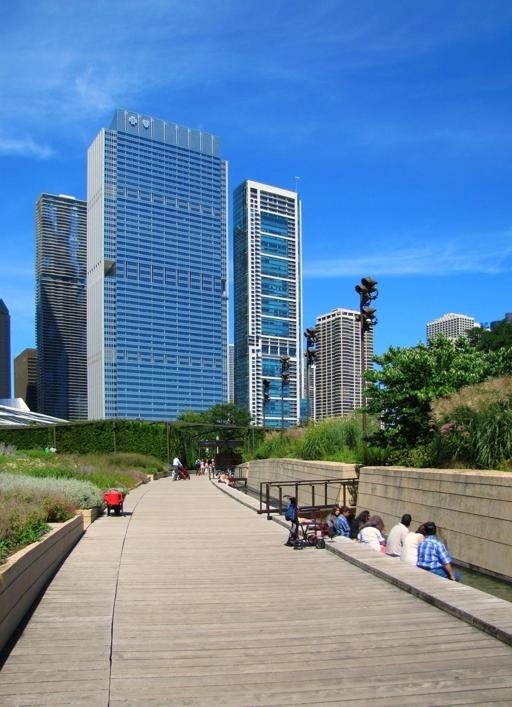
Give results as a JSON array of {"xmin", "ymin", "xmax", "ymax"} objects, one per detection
[{"xmin": 283, "ymin": 494, "xmax": 325, "ymax": 550}]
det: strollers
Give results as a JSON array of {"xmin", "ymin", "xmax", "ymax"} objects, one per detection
[{"xmin": 178, "ymin": 465, "xmax": 190, "ymax": 480}]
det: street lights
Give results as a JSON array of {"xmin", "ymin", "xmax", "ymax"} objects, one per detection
[
  {"xmin": 263, "ymin": 379, "xmax": 271, "ymax": 426},
  {"xmin": 280, "ymin": 356, "xmax": 290, "ymax": 428},
  {"xmin": 355, "ymin": 277, "xmax": 379, "ymax": 463},
  {"xmin": 304, "ymin": 327, "xmax": 319, "ymax": 424}
]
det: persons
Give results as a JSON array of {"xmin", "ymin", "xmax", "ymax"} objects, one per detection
[
  {"xmin": 45, "ymin": 445, "xmax": 57, "ymax": 454},
  {"xmin": 324, "ymin": 506, "xmax": 461, "ymax": 582},
  {"xmin": 173, "ymin": 455, "xmax": 182, "ymax": 480},
  {"xmin": 195, "ymin": 458, "xmax": 229, "ymax": 486}
]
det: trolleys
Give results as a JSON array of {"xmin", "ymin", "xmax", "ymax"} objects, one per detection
[{"xmin": 103, "ymin": 492, "xmax": 124, "ymax": 516}]
